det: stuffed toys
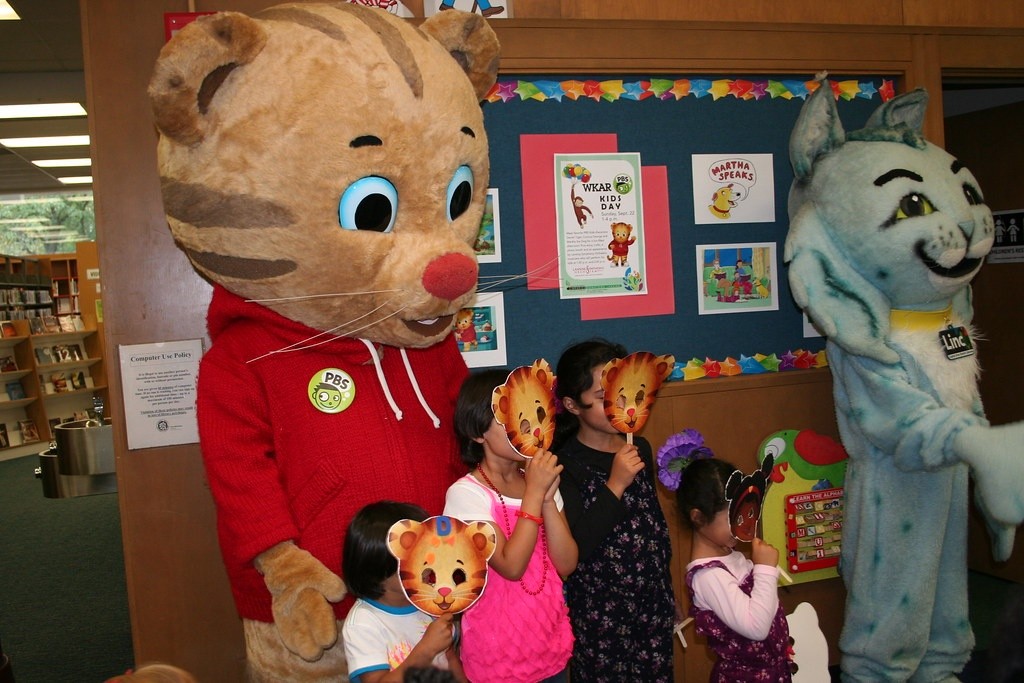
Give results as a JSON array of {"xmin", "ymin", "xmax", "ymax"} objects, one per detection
[
  {"xmin": 147, "ymin": 6, "xmax": 500, "ymax": 683},
  {"xmin": 784, "ymin": 80, "xmax": 1022, "ymax": 683}
]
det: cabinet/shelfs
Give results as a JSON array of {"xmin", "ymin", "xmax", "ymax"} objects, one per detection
[{"xmin": 0, "ymin": 241, "xmax": 114, "ymax": 464}]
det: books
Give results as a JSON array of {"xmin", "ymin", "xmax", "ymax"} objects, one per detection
[{"xmin": 2, "ymin": 283, "xmax": 100, "ymax": 454}]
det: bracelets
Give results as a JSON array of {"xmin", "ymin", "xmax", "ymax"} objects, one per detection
[{"xmin": 514, "ymin": 509, "xmax": 544, "ymax": 524}]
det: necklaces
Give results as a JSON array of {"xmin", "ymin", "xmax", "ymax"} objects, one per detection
[{"xmin": 473, "ymin": 456, "xmax": 547, "ymax": 595}]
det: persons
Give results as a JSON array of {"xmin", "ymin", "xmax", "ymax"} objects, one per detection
[
  {"xmin": 549, "ymin": 340, "xmax": 676, "ymax": 683},
  {"xmin": 437, "ymin": 366, "xmax": 577, "ymax": 683},
  {"xmin": 673, "ymin": 464, "xmax": 800, "ymax": 681},
  {"xmin": 342, "ymin": 497, "xmax": 469, "ymax": 682}
]
